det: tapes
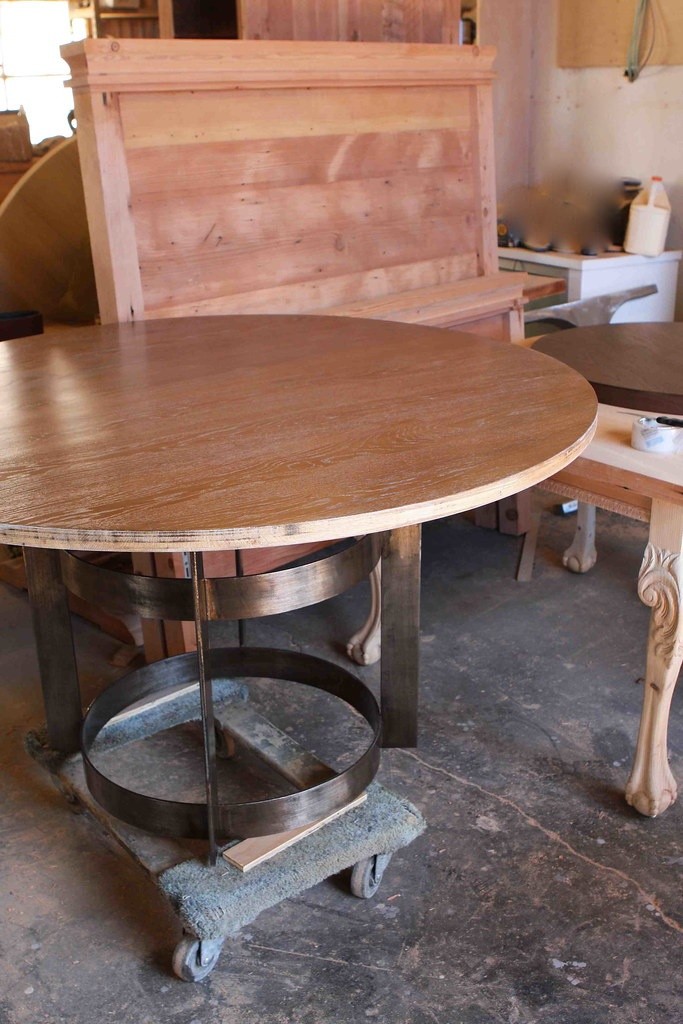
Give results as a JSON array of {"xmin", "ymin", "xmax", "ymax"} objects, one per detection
[{"xmin": 630, "ymin": 417, "xmax": 683, "ymax": 454}]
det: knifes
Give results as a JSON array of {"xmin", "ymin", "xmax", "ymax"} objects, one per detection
[{"xmin": 617, "ymin": 410, "xmax": 683, "ymax": 427}]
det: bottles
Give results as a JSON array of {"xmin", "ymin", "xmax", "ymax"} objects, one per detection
[
  {"xmin": 613, "ymin": 176, "xmax": 671, "ymax": 255},
  {"xmin": 496, "ymin": 204, "xmax": 508, "ymax": 247}
]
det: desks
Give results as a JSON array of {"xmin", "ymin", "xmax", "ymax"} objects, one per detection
[
  {"xmin": 0, "ymin": 314, "xmax": 600, "ymax": 747},
  {"xmin": 346, "ymin": 403, "xmax": 683, "ymax": 818},
  {"xmin": 524, "ymin": 322, "xmax": 683, "ymax": 412}
]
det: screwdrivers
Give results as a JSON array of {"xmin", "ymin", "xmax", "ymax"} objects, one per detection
[{"xmin": 617, "ymin": 411, "xmax": 682, "ymax": 427}]
